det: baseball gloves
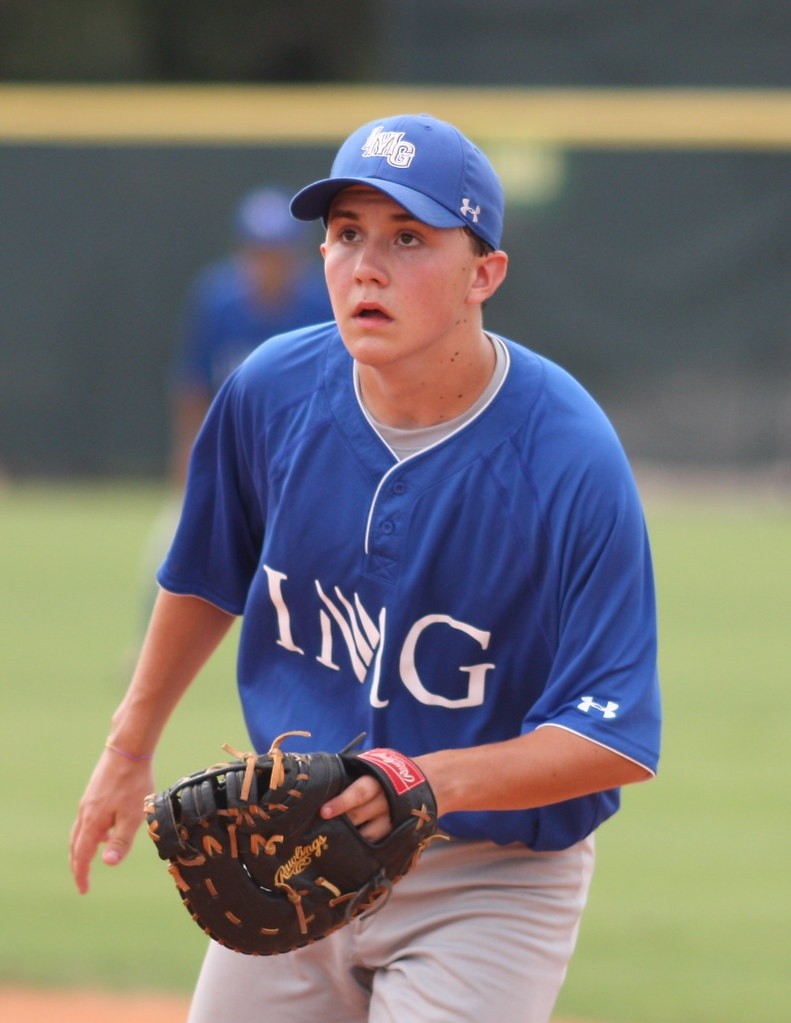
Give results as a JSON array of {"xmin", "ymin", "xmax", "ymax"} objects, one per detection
[{"xmin": 139, "ymin": 726, "xmax": 451, "ymax": 958}]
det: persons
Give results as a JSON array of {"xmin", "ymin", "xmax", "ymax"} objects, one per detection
[
  {"xmin": 70, "ymin": 113, "xmax": 661, "ymax": 1023},
  {"xmin": 169, "ymin": 190, "xmax": 335, "ymax": 487}
]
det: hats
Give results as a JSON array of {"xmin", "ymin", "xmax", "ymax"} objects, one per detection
[
  {"xmin": 290, "ymin": 112, "xmax": 502, "ymax": 252},
  {"xmin": 237, "ymin": 189, "xmax": 300, "ymax": 245}
]
